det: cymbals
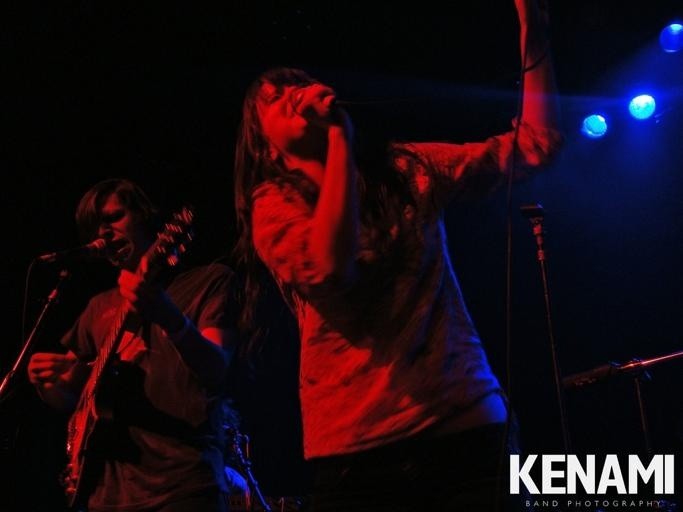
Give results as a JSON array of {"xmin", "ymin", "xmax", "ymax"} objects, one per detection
[{"xmin": 562, "ymin": 351, "xmax": 682, "ymax": 386}]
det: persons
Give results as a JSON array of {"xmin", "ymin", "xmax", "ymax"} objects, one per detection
[
  {"xmin": 22, "ymin": 173, "xmax": 260, "ymax": 512},
  {"xmin": 224, "ymin": 2, "xmax": 568, "ymax": 512}
]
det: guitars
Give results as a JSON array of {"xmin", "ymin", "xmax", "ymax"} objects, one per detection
[{"xmin": 59, "ymin": 205, "xmax": 198, "ymax": 507}]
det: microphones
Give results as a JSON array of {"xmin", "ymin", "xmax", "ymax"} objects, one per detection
[
  {"xmin": 322, "ymin": 94, "xmax": 337, "ymax": 108},
  {"xmin": 38, "ymin": 238, "xmax": 108, "ymax": 266},
  {"xmin": 561, "ymin": 362, "xmax": 620, "ymax": 389}
]
